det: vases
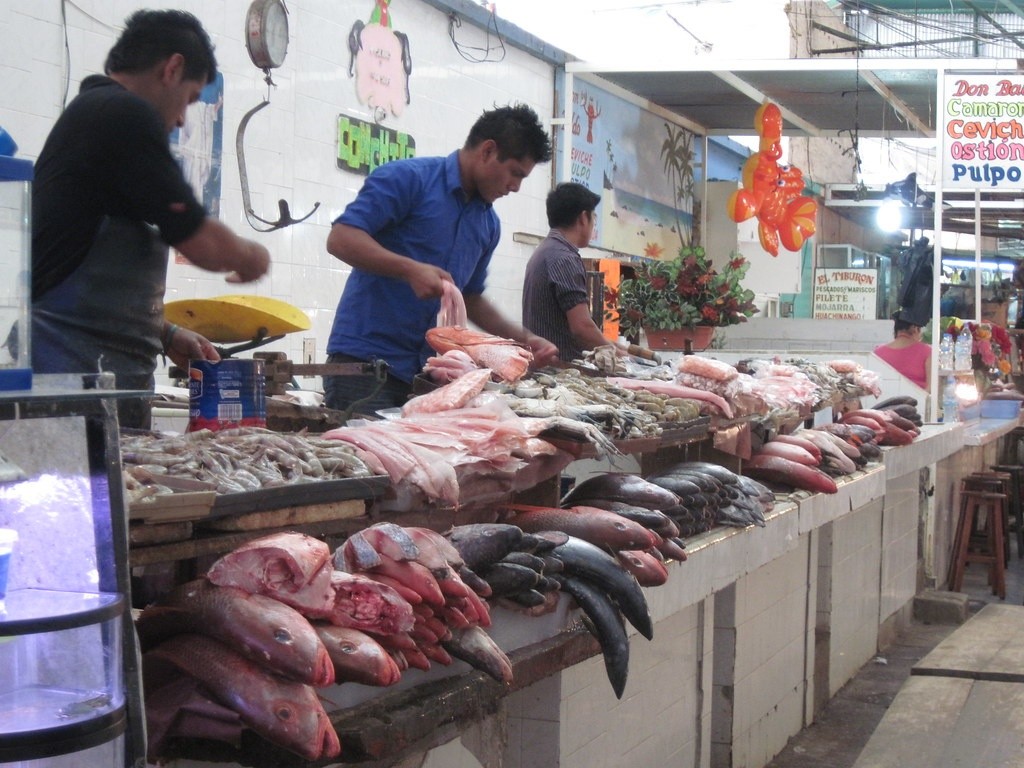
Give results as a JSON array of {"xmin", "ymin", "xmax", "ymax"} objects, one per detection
[{"xmin": 646, "ymin": 326, "xmax": 713, "ymax": 352}]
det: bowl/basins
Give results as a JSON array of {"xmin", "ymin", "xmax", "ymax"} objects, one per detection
[{"xmin": 981, "ymin": 400, "xmax": 1024, "ymax": 419}]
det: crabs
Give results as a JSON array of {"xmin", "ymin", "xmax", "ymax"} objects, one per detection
[{"xmin": 500, "ymin": 373, "xmax": 643, "ymax": 461}]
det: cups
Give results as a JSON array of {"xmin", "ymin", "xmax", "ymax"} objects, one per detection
[{"xmin": 0, "ymin": 528, "xmax": 20, "ymax": 614}]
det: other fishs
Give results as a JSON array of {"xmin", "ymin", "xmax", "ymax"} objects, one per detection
[
  {"xmin": 124, "ymin": 460, "xmax": 780, "ymax": 763},
  {"xmin": 603, "ymin": 350, "xmax": 921, "ymax": 494},
  {"xmin": 423, "ymin": 323, "xmax": 534, "ymax": 382}
]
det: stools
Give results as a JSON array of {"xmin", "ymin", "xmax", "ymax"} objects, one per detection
[{"xmin": 948, "ymin": 464, "xmax": 1024, "ymax": 599}]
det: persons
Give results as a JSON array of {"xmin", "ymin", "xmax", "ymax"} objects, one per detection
[
  {"xmin": 25, "ymin": 9, "xmax": 272, "ymax": 432},
  {"xmin": 872, "ymin": 311, "xmax": 933, "ymax": 394},
  {"xmin": 522, "ymin": 180, "xmax": 627, "ymax": 363},
  {"xmin": 317, "ymin": 100, "xmax": 559, "ymax": 424}
]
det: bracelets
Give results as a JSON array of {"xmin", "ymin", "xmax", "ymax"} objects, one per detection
[{"xmin": 162, "ymin": 322, "xmax": 177, "ymax": 365}]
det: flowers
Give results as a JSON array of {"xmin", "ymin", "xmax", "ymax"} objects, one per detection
[
  {"xmin": 605, "ymin": 242, "xmax": 760, "ymax": 338},
  {"xmin": 922, "ymin": 317, "xmax": 1011, "ymax": 375}
]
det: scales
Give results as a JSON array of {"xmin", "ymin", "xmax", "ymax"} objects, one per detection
[
  {"xmin": 235, "ymin": 0, "xmax": 319, "ymax": 232},
  {"xmin": 162, "ymin": 295, "xmax": 394, "ymax": 433}
]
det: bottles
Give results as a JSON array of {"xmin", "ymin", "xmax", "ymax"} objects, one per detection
[
  {"xmin": 955, "ymin": 336, "xmax": 967, "ymax": 371},
  {"xmin": 959, "ymin": 323, "xmax": 972, "ymax": 369},
  {"xmin": 939, "ymin": 333, "xmax": 953, "ymax": 375},
  {"xmin": 942, "ymin": 377, "xmax": 959, "ymax": 424}
]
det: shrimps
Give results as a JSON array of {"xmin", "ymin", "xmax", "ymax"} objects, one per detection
[
  {"xmin": 550, "ymin": 364, "xmax": 704, "ymax": 424},
  {"xmin": 112, "ymin": 426, "xmax": 383, "ymax": 512}
]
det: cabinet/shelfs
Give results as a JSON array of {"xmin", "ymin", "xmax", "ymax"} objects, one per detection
[{"xmin": 128, "ymin": 385, "xmax": 1017, "ymax": 768}]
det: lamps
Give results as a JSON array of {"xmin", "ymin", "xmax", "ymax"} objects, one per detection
[{"xmin": 871, "ymin": 195, "xmax": 911, "ymax": 234}]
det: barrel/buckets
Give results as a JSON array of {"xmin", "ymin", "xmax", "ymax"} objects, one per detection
[{"xmin": 187, "ymin": 358, "xmax": 266, "ymax": 434}]
